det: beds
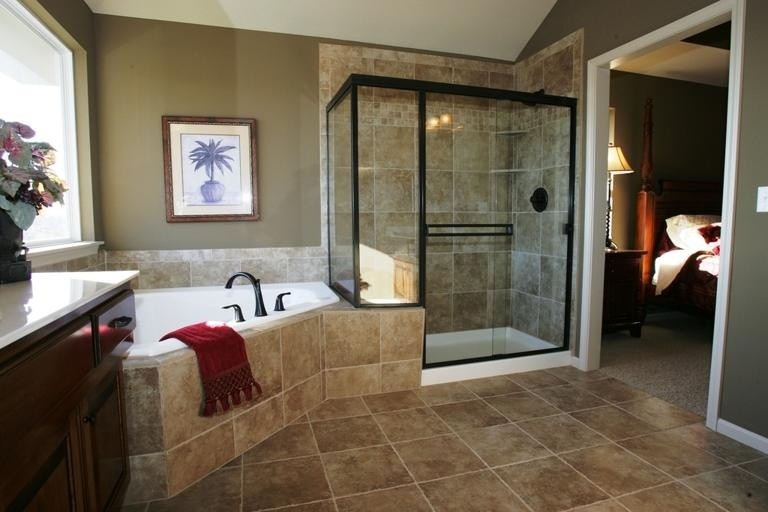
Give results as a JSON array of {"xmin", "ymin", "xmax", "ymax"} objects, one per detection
[{"xmin": 636, "ymin": 97, "xmax": 720, "ymax": 327}]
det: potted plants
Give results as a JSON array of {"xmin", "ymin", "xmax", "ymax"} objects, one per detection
[{"xmin": 189, "ymin": 139, "xmax": 236, "ymax": 204}]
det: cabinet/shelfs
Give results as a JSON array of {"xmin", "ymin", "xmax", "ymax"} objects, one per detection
[{"xmin": 0, "ymin": 282, "xmax": 137, "ymax": 510}]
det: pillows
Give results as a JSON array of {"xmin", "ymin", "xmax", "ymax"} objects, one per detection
[{"xmin": 660, "ymin": 213, "xmax": 720, "ymax": 256}]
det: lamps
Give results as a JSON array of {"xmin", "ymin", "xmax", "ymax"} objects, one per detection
[{"xmin": 605, "ymin": 143, "xmax": 635, "ymax": 250}]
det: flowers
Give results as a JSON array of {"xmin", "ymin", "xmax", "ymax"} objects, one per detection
[{"xmin": 0, "ymin": 120, "xmax": 66, "ymax": 228}]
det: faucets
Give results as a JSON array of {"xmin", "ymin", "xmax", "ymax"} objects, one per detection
[{"xmin": 225, "ymin": 271, "xmax": 266, "ymax": 317}]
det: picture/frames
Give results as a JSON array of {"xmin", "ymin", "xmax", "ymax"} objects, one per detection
[{"xmin": 161, "ymin": 116, "xmax": 259, "ymax": 222}]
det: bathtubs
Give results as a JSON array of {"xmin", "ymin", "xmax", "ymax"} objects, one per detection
[{"xmin": 110, "ymin": 279, "xmax": 341, "ymax": 358}]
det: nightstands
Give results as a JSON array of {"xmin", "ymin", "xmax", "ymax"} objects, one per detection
[{"xmin": 603, "ymin": 247, "xmax": 648, "ymax": 338}]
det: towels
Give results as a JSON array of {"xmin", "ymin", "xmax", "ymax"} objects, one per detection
[{"xmin": 160, "ymin": 319, "xmax": 261, "ymax": 417}]
